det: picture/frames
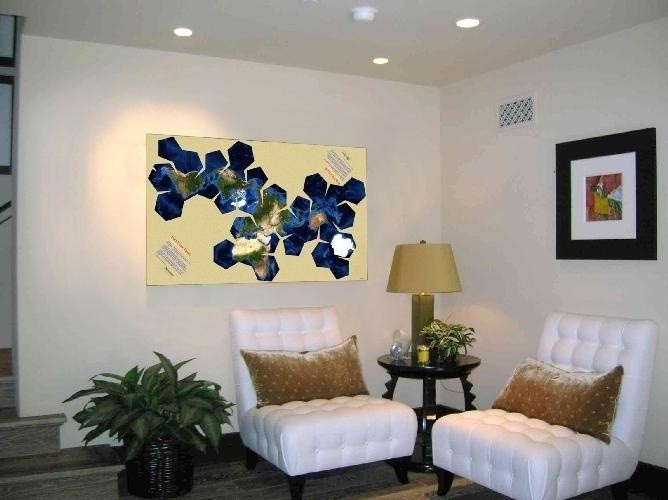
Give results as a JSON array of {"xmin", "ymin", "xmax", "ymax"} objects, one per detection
[{"xmin": 553, "ymin": 125, "xmax": 657, "ymax": 262}]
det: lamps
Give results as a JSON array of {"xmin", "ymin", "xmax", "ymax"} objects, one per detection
[{"xmin": 384, "ymin": 242, "xmax": 463, "ymax": 358}]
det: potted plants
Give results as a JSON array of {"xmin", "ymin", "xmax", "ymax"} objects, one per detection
[{"xmin": 68, "ymin": 350, "xmax": 233, "ymax": 500}]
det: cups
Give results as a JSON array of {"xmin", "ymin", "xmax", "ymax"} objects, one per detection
[{"xmin": 417, "ymin": 345, "xmax": 430, "ymax": 366}]
